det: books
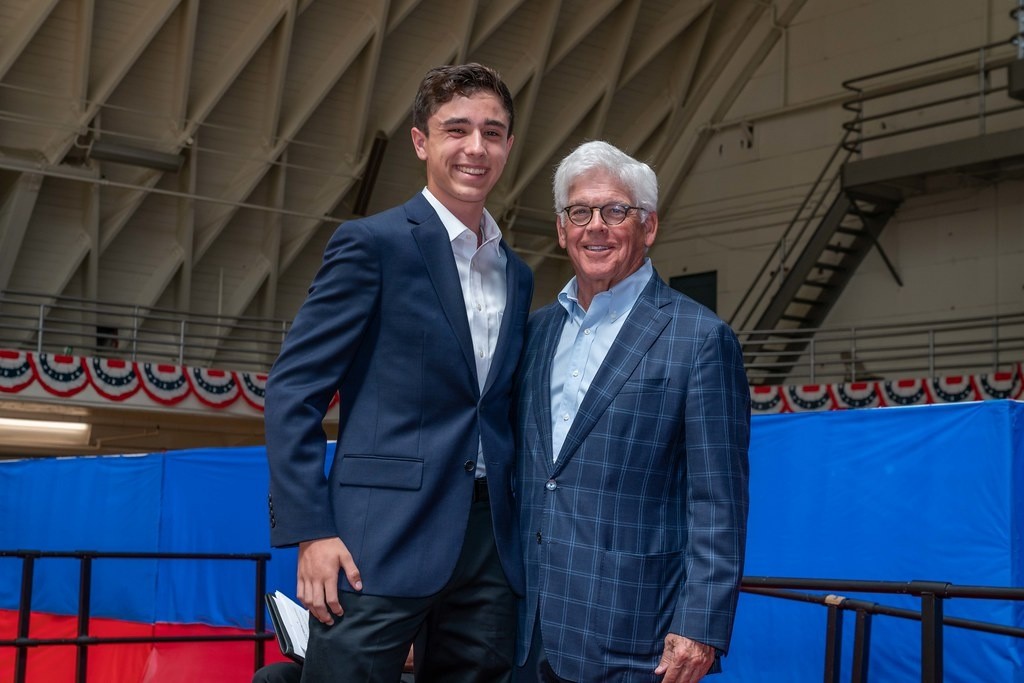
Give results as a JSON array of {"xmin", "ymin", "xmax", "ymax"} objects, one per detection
[{"xmin": 265, "ymin": 590, "xmax": 308, "ymax": 663}]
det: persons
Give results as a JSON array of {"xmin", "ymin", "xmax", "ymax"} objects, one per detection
[
  {"xmin": 252, "ymin": 643, "xmax": 413, "ymax": 683},
  {"xmin": 518, "ymin": 141, "xmax": 749, "ymax": 683},
  {"xmin": 265, "ymin": 63, "xmax": 536, "ymax": 683}
]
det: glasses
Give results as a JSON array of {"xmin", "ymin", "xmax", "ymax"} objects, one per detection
[{"xmin": 563, "ymin": 204, "xmax": 648, "ymax": 227}]
